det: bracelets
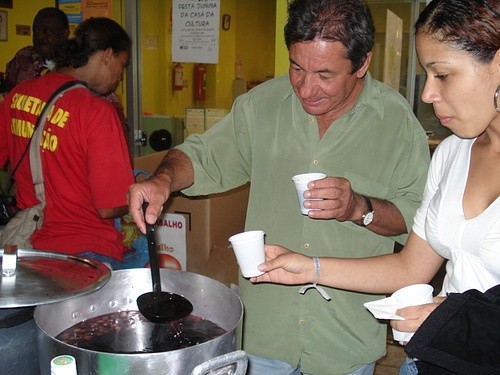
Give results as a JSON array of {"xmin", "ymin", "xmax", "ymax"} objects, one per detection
[{"xmin": 299, "ymin": 256, "xmax": 331, "ymax": 301}]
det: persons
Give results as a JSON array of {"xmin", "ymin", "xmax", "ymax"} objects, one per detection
[
  {"xmin": 0, "ymin": 6, "xmax": 129, "ymax": 230},
  {"xmin": 6, "ymin": 16, "xmax": 161, "ymax": 272},
  {"xmin": 249, "ymin": 0, "xmax": 500, "ymax": 375},
  {"xmin": 126, "ymin": 0, "xmax": 428, "ymax": 375}
]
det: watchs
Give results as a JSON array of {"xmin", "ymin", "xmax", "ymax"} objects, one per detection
[{"xmin": 357, "ymin": 194, "xmax": 374, "ymax": 229}]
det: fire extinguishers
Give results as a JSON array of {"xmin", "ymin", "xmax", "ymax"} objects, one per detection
[
  {"xmin": 194, "ymin": 64, "xmax": 207, "ymax": 102},
  {"xmin": 174, "ymin": 63, "xmax": 183, "ymax": 90}
]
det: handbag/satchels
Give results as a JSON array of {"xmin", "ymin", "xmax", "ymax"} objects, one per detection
[
  {"xmin": 403, "ymin": 285, "xmax": 500, "ymax": 375},
  {"xmin": 0, "ymin": 207, "xmax": 44, "ymax": 251}
]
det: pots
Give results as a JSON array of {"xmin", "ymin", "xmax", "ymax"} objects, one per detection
[{"xmin": 32, "ymin": 267, "xmax": 249, "ymax": 375}]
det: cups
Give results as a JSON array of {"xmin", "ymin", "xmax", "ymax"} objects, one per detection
[
  {"xmin": 291, "ymin": 172, "xmax": 328, "ymax": 216},
  {"xmin": 228, "ymin": 230, "xmax": 268, "ymax": 278},
  {"xmin": 391, "ymin": 284, "xmax": 434, "ymax": 346}
]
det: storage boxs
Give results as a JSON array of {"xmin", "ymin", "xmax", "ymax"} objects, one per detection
[{"xmin": 129, "ymin": 149, "xmax": 249, "ymax": 275}]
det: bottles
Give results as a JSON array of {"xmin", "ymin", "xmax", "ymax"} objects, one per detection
[{"xmin": 50, "ymin": 354, "xmax": 77, "ymax": 375}]
